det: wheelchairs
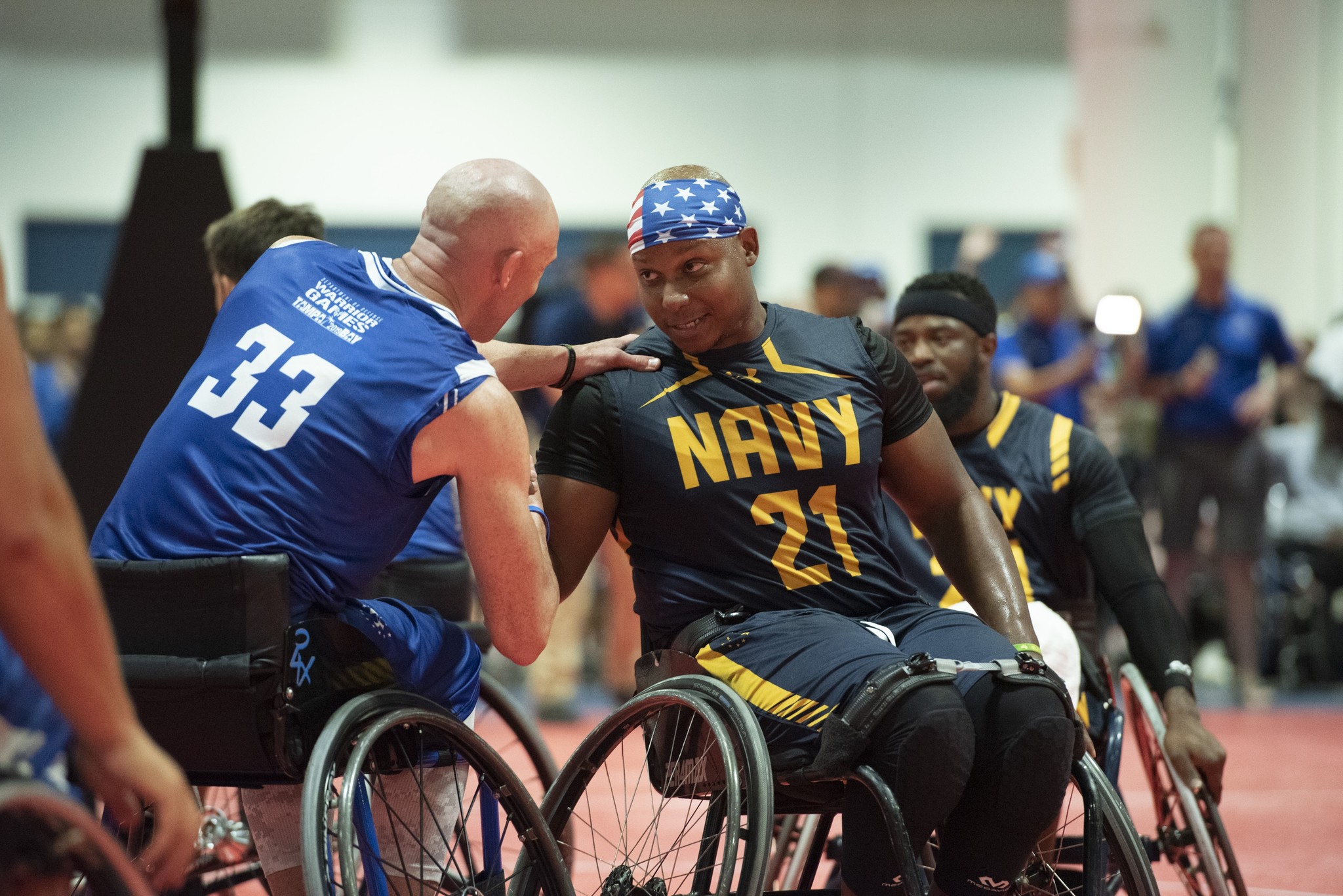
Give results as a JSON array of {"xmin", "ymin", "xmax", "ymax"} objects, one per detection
[
  {"xmin": 0, "ymin": 555, "xmax": 580, "ymax": 896},
  {"xmin": 676, "ymin": 664, "xmax": 1252, "ymax": 896},
  {"xmin": 504, "ymin": 623, "xmax": 1170, "ymax": 896}
]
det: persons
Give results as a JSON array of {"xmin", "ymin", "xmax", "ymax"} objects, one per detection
[
  {"xmin": 0, "ymin": 201, "xmax": 1343, "ymax": 710},
  {"xmin": 888, "ymin": 271, "xmax": 1225, "ymax": 807},
  {"xmin": 93, "ymin": 161, "xmax": 660, "ymax": 896},
  {"xmin": 537, "ymin": 164, "xmax": 1100, "ymax": 896},
  {"xmin": 0, "ymin": 246, "xmax": 196, "ymax": 889}
]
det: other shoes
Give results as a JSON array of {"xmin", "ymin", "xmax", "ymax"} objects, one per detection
[{"xmin": 1238, "ymin": 673, "xmax": 1277, "ymax": 704}]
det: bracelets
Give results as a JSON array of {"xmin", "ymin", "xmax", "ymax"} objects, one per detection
[
  {"xmin": 528, "ymin": 506, "xmax": 549, "ymax": 543},
  {"xmin": 547, "ymin": 343, "xmax": 575, "ymax": 389},
  {"xmin": 1013, "ymin": 643, "xmax": 1043, "ymax": 656}
]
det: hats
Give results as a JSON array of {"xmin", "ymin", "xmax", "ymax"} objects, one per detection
[{"xmin": 1012, "ymin": 248, "xmax": 1060, "ymax": 287}]
